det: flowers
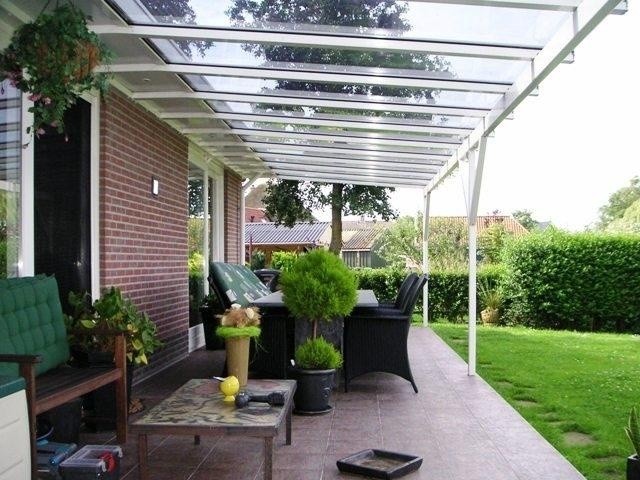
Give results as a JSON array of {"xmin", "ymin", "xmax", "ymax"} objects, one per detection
[
  {"xmin": 215, "ymin": 303, "xmax": 267, "ymax": 355},
  {"xmin": 0, "ymin": 2, "xmax": 119, "ymax": 148}
]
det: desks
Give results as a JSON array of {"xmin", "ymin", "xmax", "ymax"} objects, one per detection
[{"xmin": 251, "ymin": 288, "xmax": 380, "ymax": 388}]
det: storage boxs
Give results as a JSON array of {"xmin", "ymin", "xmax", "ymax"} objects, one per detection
[
  {"xmin": 59, "ymin": 444, "xmax": 124, "ymax": 480},
  {"xmin": 35, "ymin": 440, "xmax": 77, "ymax": 480}
]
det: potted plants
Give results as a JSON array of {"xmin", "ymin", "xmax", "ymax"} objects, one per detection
[
  {"xmin": 276, "ymin": 245, "xmax": 362, "ymax": 416},
  {"xmin": 624, "ymin": 407, "xmax": 640, "ymax": 480},
  {"xmin": 476, "ymin": 274, "xmax": 506, "ymax": 325},
  {"xmin": 63, "ymin": 286, "xmax": 166, "ymax": 432}
]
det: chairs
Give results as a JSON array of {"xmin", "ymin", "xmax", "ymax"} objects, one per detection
[
  {"xmin": 199, "ymin": 261, "xmax": 280, "ymax": 352},
  {"xmin": 344, "ymin": 272, "xmax": 427, "ymax": 394},
  {"xmin": 0, "ymin": 274, "xmax": 126, "ymax": 480}
]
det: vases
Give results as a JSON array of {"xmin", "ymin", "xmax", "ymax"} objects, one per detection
[
  {"xmin": 37, "ymin": 37, "xmax": 100, "ymax": 85},
  {"xmin": 225, "ymin": 336, "xmax": 250, "ymax": 386}
]
